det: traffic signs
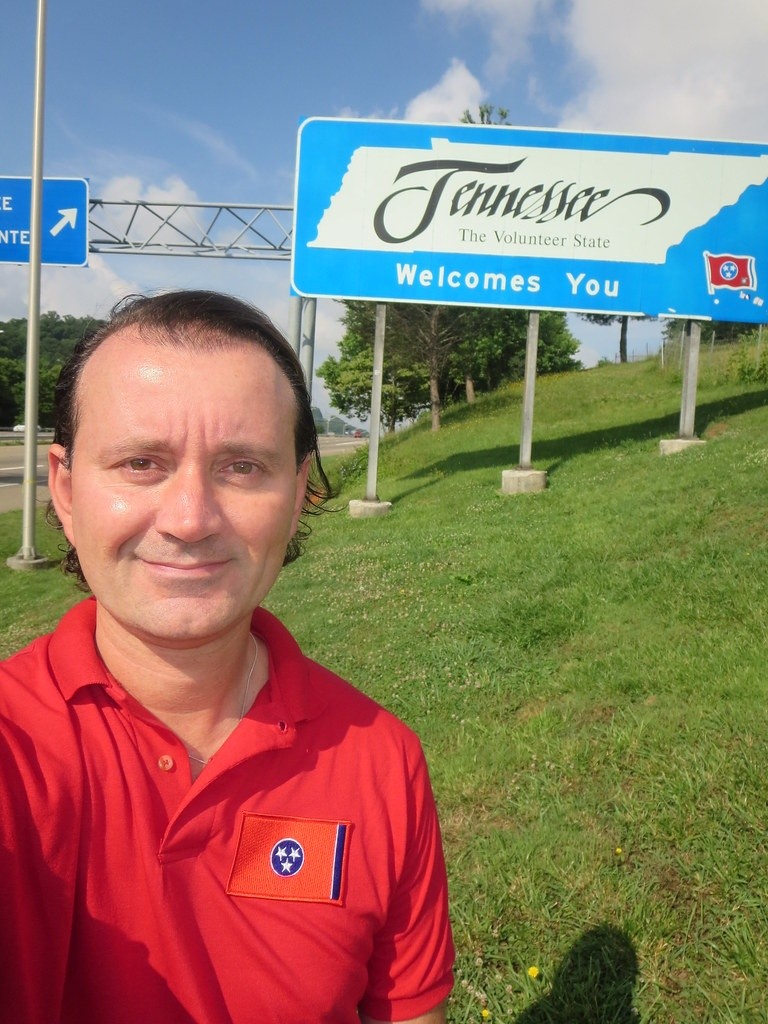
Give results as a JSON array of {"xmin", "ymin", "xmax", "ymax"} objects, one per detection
[{"xmin": 0, "ymin": 175, "xmax": 89, "ymax": 268}]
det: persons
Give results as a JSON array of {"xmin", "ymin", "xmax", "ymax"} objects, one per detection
[{"xmin": 0, "ymin": 290, "xmax": 456, "ymax": 1024}]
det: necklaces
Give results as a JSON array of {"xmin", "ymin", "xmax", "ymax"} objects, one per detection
[{"xmin": 184, "ymin": 630, "xmax": 258, "ymax": 764}]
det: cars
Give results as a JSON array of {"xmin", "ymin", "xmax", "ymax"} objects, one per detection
[
  {"xmin": 13, "ymin": 422, "xmax": 42, "ymax": 433},
  {"xmin": 354, "ymin": 431, "xmax": 362, "ymax": 438}
]
